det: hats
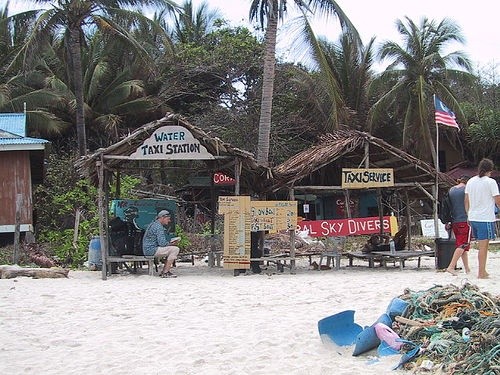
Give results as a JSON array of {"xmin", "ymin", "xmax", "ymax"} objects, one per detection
[{"xmin": 156, "ymin": 209, "xmax": 169, "ymax": 217}]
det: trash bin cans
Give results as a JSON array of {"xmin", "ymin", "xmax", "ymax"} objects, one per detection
[{"xmin": 435, "ymin": 238, "xmax": 458, "ymax": 269}]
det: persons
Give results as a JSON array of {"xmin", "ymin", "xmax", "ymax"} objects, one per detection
[
  {"xmin": 463, "ymin": 159, "xmax": 500, "ymax": 279},
  {"xmin": 366, "ymin": 224, "xmax": 407, "ymax": 252},
  {"xmin": 240, "ymin": 231, "xmax": 264, "ymax": 274},
  {"xmin": 142, "ymin": 209, "xmax": 180, "ymax": 278},
  {"xmin": 446, "ymin": 175, "xmax": 472, "ymax": 275}
]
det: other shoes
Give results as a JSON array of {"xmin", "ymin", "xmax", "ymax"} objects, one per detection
[{"xmin": 161, "ymin": 271, "xmax": 177, "ymax": 278}]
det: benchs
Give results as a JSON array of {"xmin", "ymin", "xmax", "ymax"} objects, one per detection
[
  {"xmin": 248, "ymin": 247, "xmax": 437, "ymax": 274},
  {"xmin": 105, "ymin": 250, "xmax": 225, "ymax": 278}
]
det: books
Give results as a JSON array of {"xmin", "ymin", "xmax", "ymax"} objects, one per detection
[{"xmin": 170, "ymin": 236, "xmax": 181, "ymax": 243}]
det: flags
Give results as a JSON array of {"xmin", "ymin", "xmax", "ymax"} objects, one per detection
[{"xmin": 433, "ymin": 94, "xmax": 460, "ymax": 132}]
